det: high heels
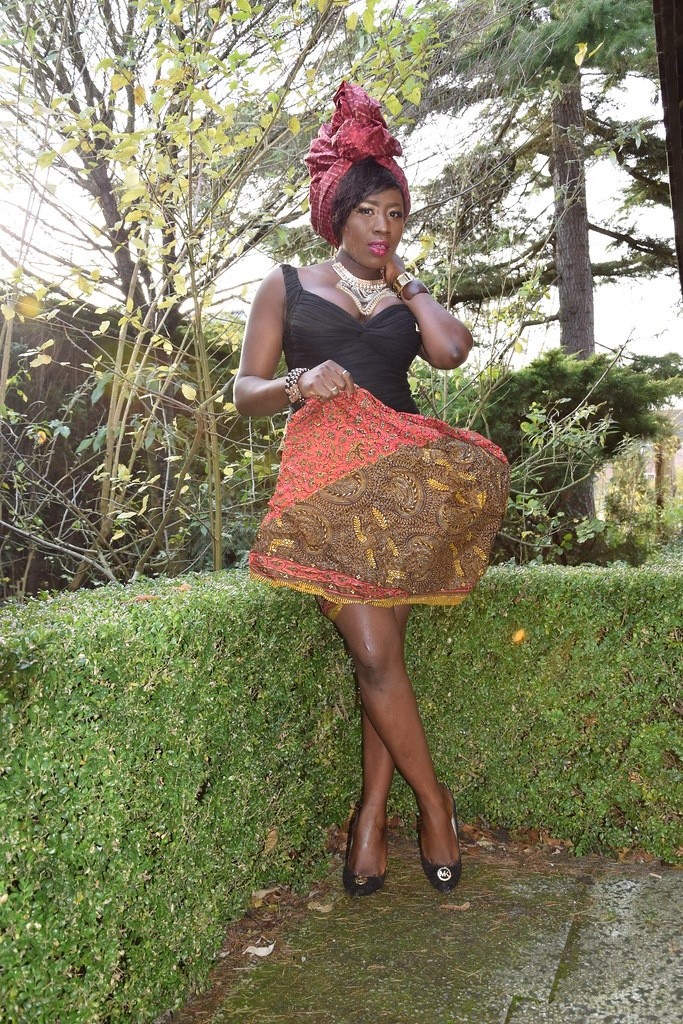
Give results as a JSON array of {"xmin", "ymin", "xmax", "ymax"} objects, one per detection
[
  {"xmin": 416, "ymin": 785, "xmax": 461, "ymax": 893},
  {"xmin": 342, "ymin": 806, "xmax": 387, "ymax": 898}
]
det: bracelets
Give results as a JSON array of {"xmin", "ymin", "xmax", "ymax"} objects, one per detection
[
  {"xmin": 403, "ymin": 280, "xmax": 429, "ymax": 300},
  {"xmin": 286, "ymin": 368, "xmax": 310, "ymax": 409},
  {"xmin": 393, "ymin": 272, "xmax": 415, "ymax": 298}
]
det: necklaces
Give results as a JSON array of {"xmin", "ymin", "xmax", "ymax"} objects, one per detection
[{"xmin": 332, "ymin": 256, "xmax": 397, "ymax": 315}]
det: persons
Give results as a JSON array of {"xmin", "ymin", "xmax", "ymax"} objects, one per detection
[{"xmin": 232, "ymin": 82, "xmax": 507, "ymax": 895}]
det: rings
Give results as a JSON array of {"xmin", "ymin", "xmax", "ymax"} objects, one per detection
[
  {"xmin": 342, "ymin": 370, "xmax": 347, "ymax": 374},
  {"xmin": 331, "ymin": 386, "xmax": 337, "ymax": 391}
]
